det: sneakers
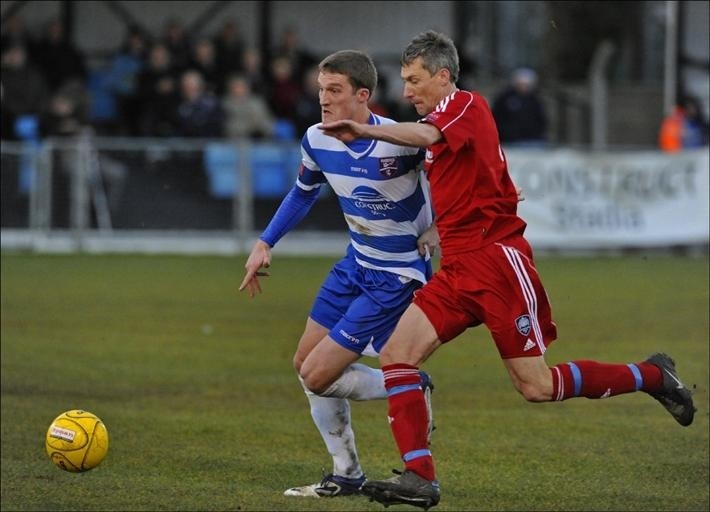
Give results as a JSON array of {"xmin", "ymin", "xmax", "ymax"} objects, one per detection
[
  {"xmin": 419, "ymin": 371, "xmax": 436, "ymax": 445},
  {"xmin": 646, "ymin": 352, "xmax": 697, "ymax": 426},
  {"xmin": 283, "ymin": 473, "xmax": 368, "ymax": 498},
  {"xmin": 361, "ymin": 471, "xmax": 441, "ymax": 512}
]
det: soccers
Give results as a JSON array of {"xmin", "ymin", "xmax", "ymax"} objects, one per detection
[{"xmin": 46, "ymin": 410, "xmax": 109, "ymax": 473}]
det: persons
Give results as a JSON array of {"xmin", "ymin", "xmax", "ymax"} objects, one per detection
[
  {"xmin": 315, "ymin": 26, "xmax": 699, "ymax": 511},
  {"xmin": 235, "ymin": 47, "xmax": 441, "ymax": 501},
  {"xmin": 684, "ymin": 95, "xmax": 710, "ymax": 150},
  {"xmin": 1, "ymin": 8, "xmax": 401, "ymax": 232},
  {"xmin": 490, "ymin": 65, "xmax": 551, "ymax": 145},
  {"xmin": 656, "ymin": 92, "xmax": 705, "ymax": 151}
]
